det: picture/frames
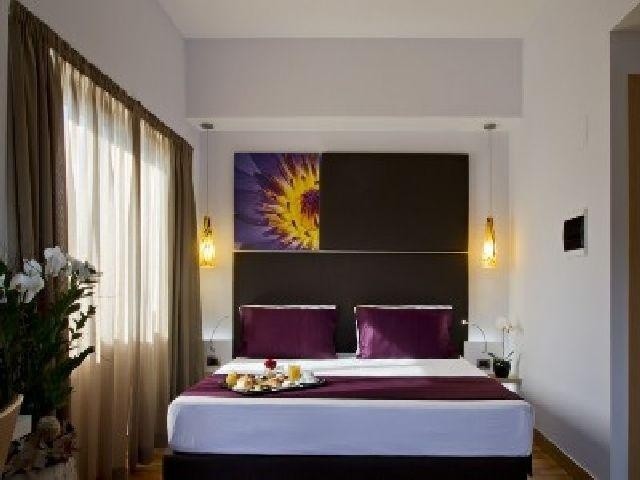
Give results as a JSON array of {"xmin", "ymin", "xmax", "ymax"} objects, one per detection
[{"xmin": 477, "ymin": 360, "xmax": 490, "ymax": 370}]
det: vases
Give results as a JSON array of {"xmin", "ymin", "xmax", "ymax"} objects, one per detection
[{"xmin": 493, "ymin": 360, "xmax": 511, "ymax": 378}]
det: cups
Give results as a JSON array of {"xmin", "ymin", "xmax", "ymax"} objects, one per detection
[
  {"xmin": 288, "ymin": 363, "xmax": 301, "ymax": 383},
  {"xmin": 227, "ymin": 371, "xmax": 237, "ymax": 390},
  {"xmin": 303, "ymin": 370, "xmax": 314, "ymax": 380}
]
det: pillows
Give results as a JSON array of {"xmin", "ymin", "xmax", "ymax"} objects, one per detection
[
  {"xmin": 353, "ymin": 303, "xmax": 458, "ymax": 361},
  {"xmin": 238, "ymin": 303, "xmax": 338, "ymax": 359}
]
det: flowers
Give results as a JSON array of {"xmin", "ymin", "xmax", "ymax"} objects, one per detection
[
  {"xmin": 489, "ymin": 316, "xmax": 514, "ymax": 361},
  {"xmin": 0, "ymin": 245, "xmax": 102, "ymax": 423}
]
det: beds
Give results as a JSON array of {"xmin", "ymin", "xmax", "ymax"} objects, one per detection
[{"xmin": 163, "ymin": 249, "xmax": 535, "ymax": 478}]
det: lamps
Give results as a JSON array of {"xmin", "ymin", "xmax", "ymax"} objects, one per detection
[
  {"xmin": 461, "ymin": 319, "xmax": 491, "ymax": 358},
  {"xmin": 207, "ymin": 314, "xmax": 229, "ymax": 359},
  {"xmin": 199, "ymin": 123, "xmax": 217, "ymax": 268},
  {"xmin": 482, "ymin": 124, "xmax": 498, "ymax": 269}
]
